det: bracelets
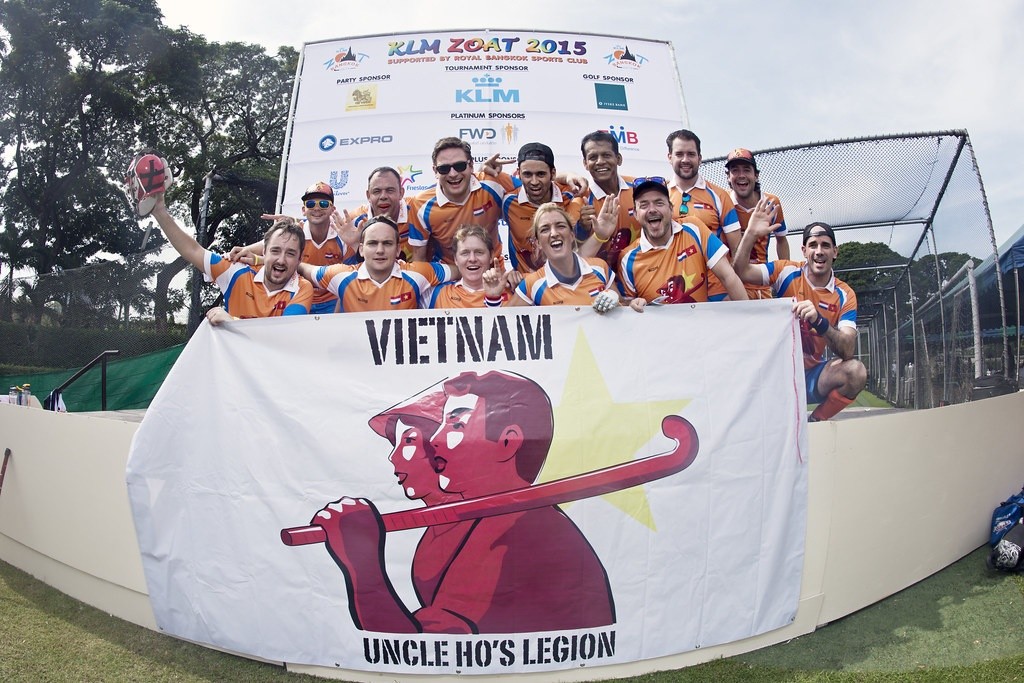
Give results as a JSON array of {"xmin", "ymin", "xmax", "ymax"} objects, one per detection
[
  {"xmin": 255, "ymin": 256, "xmax": 258, "ymax": 265},
  {"xmin": 594, "ymin": 232, "xmax": 610, "ymax": 243},
  {"xmin": 485, "ymin": 295, "xmax": 502, "ymax": 307},
  {"xmin": 808, "ymin": 309, "xmax": 829, "ymax": 334}
]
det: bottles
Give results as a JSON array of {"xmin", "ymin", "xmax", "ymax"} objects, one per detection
[{"xmin": 9, "ymin": 384, "xmax": 31, "ymax": 406}]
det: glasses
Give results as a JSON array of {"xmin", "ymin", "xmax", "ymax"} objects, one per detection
[
  {"xmin": 305, "ymin": 200, "xmax": 333, "ymax": 210},
  {"xmin": 632, "ymin": 177, "xmax": 668, "ymax": 191},
  {"xmin": 435, "ymin": 160, "xmax": 471, "ymax": 175}
]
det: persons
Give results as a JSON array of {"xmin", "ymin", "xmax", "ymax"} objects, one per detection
[
  {"xmin": 418, "ymin": 225, "xmax": 530, "ymax": 309},
  {"xmin": 573, "ymin": 130, "xmax": 644, "ymax": 271},
  {"xmin": 482, "ymin": 203, "xmax": 627, "ymax": 312},
  {"xmin": 724, "ymin": 149, "xmax": 791, "ymax": 300},
  {"xmin": 665, "ymin": 129, "xmax": 741, "ymax": 301},
  {"xmin": 618, "ymin": 182, "xmax": 749, "ymax": 312},
  {"xmin": 732, "ymin": 197, "xmax": 867, "ymax": 422},
  {"xmin": 230, "ymin": 215, "xmax": 523, "ymax": 313},
  {"xmin": 125, "ymin": 147, "xmax": 313, "ymax": 326},
  {"xmin": 221, "ymin": 136, "xmax": 589, "ymax": 261}
]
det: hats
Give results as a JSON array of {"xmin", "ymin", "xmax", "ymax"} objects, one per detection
[
  {"xmin": 633, "ymin": 178, "xmax": 669, "ymax": 202},
  {"xmin": 803, "ymin": 222, "xmax": 836, "ymax": 262},
  {"xmin": 724, "ymin": 148, "xmax": 756, "ymax": 169},
  {"xmin": 517, "ymin": 143, "xmax": 554, "ymax": 169},
  {"xmin": 302, "ymin": 182, "xmax": 334, "ymax": 203}
]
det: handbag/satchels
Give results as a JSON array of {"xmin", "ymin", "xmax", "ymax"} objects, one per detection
[{"xmin": 988, "ymin": 489, "xmax": 1024, "ymax": 570}]
